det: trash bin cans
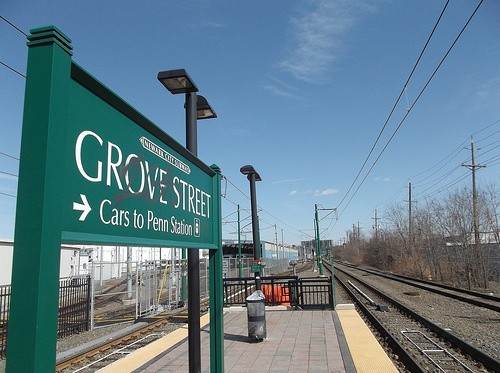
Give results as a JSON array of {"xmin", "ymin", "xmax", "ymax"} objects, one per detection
[{"xmin": 246, "ymin": 296, "xmax": 267, "ymax": 343}]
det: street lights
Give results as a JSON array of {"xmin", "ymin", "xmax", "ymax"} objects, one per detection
[
  {"xmin": 156, "ymin": 68, "xmax": 218, "ymax": 373},
  {"xmin": 240, "ymin": 164, "xmax": 263, "ymax": 290}
]
